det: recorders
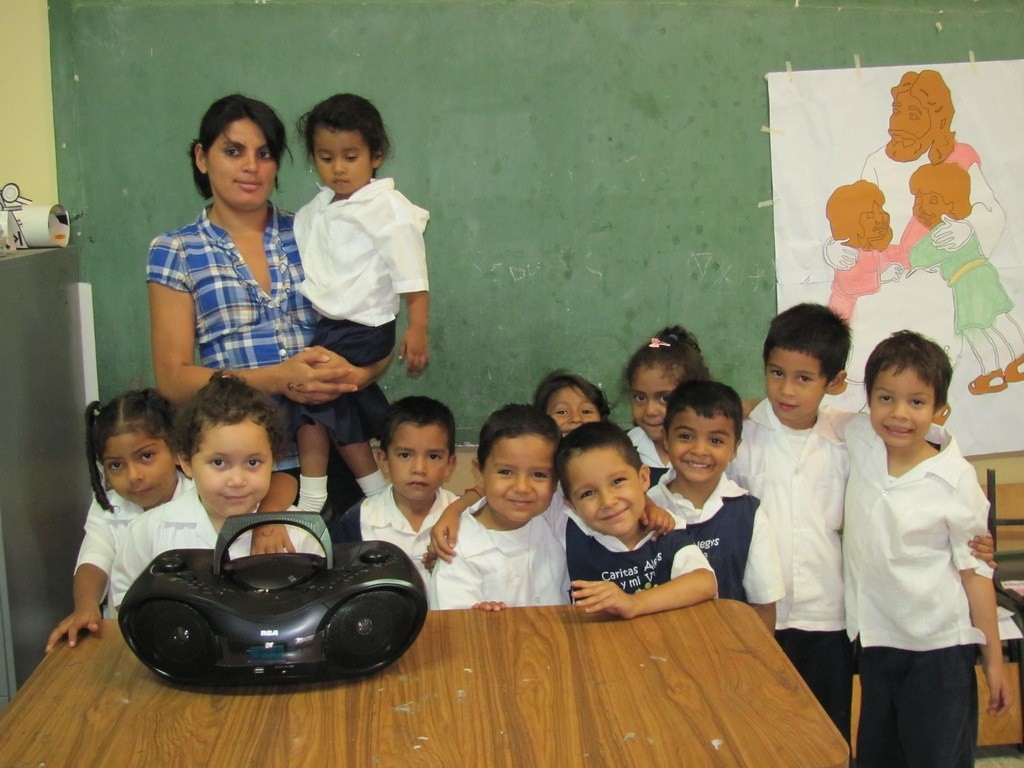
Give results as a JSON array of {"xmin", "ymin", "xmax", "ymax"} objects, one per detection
[{"xmin": 118, "ymin": 510, "xmax": 429, "ymax": 686}]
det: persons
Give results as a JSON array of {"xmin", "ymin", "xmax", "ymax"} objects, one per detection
[
  {"xmin": 142, "ymin": 93, "xmax": 433, "ymax": 538},
  {"xmin": 43, "ymin": 368, "xmax": 329, "ymax": 656},
  {"xmin": 358, "ymin": 303, "xmax": 1012, "ymax": 768}
]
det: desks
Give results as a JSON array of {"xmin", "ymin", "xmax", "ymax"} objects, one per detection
[{"xmin": 0, "ymin": 598, "xmax": 850, "ymax": 768}]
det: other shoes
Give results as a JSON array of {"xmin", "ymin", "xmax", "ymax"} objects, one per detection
[{"xmin": 317, "ymin": 496, "xmax": 344, "ymax": 529}]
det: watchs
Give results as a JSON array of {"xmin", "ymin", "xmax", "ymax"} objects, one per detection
[{"xmin": 464, "ymin": 484, "xmax": 484, "ymax": 497}]
